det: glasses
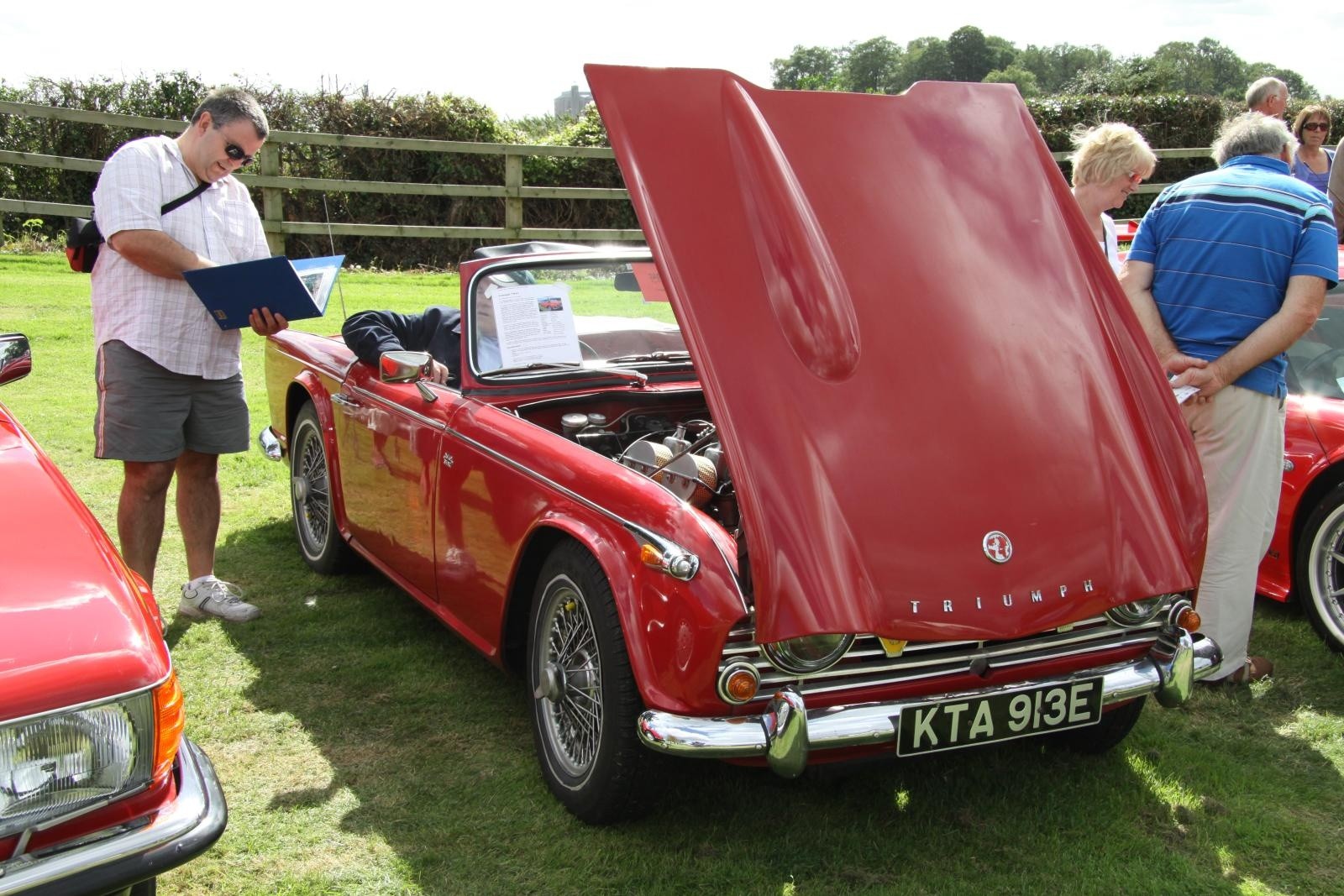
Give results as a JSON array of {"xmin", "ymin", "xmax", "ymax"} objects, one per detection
[
  {"xmin": 1274, "ymin": 96, "xmax": 1291, "ymax": 102},
  {"xmin": 1127, "ymin": 170, "xmax": 1142, "ymax": 184},
  {"xmin": 213, "ymin": 122, "xmax": 255, "ymax": 166},
  {"xmin": 1302, "ymin": 122, "xmax": 1329, "ymax": 132}
]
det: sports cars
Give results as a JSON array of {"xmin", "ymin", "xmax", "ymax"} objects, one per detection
[
  {"xmin": 260, "ymin": 64, "xmax": 1226, "ymax": 828},
  {"xmin": 1111, "ymin": 220, "xmax": 1344, "ymax": 659}
]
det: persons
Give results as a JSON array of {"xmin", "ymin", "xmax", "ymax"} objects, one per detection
[
  {"xmin": 1067, "ymin": 120, "xmax": 1160, "ymax": 281},
  {"xmin": 66, "ymin": 86, "xmax": 291, "ymax": 638},
  {"xmin": 341, "ymin": 270, "xmax": 535, "ymax": 391},
  {"xmin": 1245, "ymin": 77, "xmax": 1344, "ymax": 247},
  {"xmin": 1117, "ymin": 113, "xmax": 1337, "ymax": 688}
]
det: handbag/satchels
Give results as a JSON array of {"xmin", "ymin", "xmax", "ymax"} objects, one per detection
[{"xmin": 66, "ymin": 217, "xmax": 101, "ymax": 272}]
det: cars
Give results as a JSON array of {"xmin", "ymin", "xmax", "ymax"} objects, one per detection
[{"xmin": 0, "ymin": 331, "xmax": 230, "ymax": 896}]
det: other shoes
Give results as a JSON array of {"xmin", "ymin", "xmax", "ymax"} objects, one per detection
[{"xmin": 1197, "ymin": 655, "xmax": 1274, "ymax": 684}]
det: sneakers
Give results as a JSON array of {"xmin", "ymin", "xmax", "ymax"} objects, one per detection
[{"xmin": 179, "ymin": 579, "xmax": 261, "ymax": 621}]
